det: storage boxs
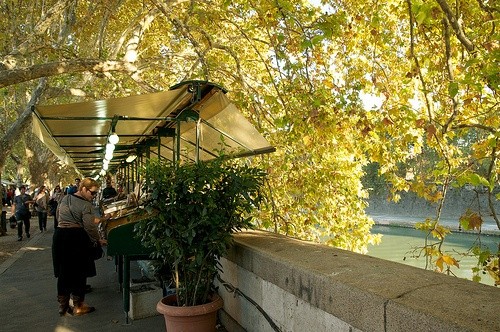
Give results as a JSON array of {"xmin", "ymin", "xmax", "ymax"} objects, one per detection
[{"xmin": 128, "ymin": 282, "xmax": 163, "ymax": 320}]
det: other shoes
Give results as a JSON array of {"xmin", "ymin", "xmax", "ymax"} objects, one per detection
[
  {"xmin": 40, "ymin": 230, "xmax": 43, "ymax": 233},
  {"xmin": 26, "ymin": 233, "xmax": 30, "ymax": 238},
  {"xmin": 18, "ymin": 237, "xmax": 22, "ymax": 241},
  {"xmin": 86, "ymin": 284, "xmax": 92, "ymax": 293}
]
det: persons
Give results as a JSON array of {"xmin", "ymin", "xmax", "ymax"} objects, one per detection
[
  {"xmin": 102, "ymin": 179, "xmax": 123, "ymax": 199},
  {"xmin": 11, "ymin": 185, "xmax": 34, "ymax": 241},
  {"xmin": 35, "ymin": 186, "xmax": 47, "ymax": 233},
  {"xmin": 53, "ymin": 178, "xmax": 111, "ymax": 294},
  {"xmin": 52, "ymin": 177, "xmax": 107, "ymax": 316},
  {"xmin": 2, "ymin": 185, "xmax": 15, "ymax": 207}
]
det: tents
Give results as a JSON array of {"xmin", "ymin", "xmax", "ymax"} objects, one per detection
[{"xmin": 1, "ymin": 180, "xmax": 16, "ymax": 192}]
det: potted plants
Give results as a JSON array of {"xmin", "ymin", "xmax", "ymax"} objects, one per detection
[{"xmin": 129, "ymin": 136, "xmax": 269, "ymax": 332}]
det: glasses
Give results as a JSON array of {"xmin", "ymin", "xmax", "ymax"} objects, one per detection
[{"xmin": 88, "ymin": 189, "xmax": 97, "ymax": 195}]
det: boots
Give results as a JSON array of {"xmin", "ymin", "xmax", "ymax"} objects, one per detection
[
  {"xmin": 56, "ymin": 295, "xmax": 74, "ymax": 315},
  {"xmin": 72, "ymin": 296, "xmax": 96, "ymax": 315}
]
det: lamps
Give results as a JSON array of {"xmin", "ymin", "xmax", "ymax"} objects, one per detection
[{"xmin": 100, "ymin": 124, "xmax": 119, "ymax": 176}]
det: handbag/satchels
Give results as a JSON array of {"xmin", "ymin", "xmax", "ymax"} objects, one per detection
[
  {"xmin": 9, "ymin": 216, "xmax": 17, "ymax": 229},
  {"xmin": 90, "ymin": 241, "xmax": 102, "ymax": 260},
  {"xmin": 47, "ymin": 199, "xmax": 58, "ymax": 216}
]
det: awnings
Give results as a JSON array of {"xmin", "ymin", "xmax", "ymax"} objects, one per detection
[{"xmin": 29, "ymin": 80, "xmax": 277, "ymax": 179}]
